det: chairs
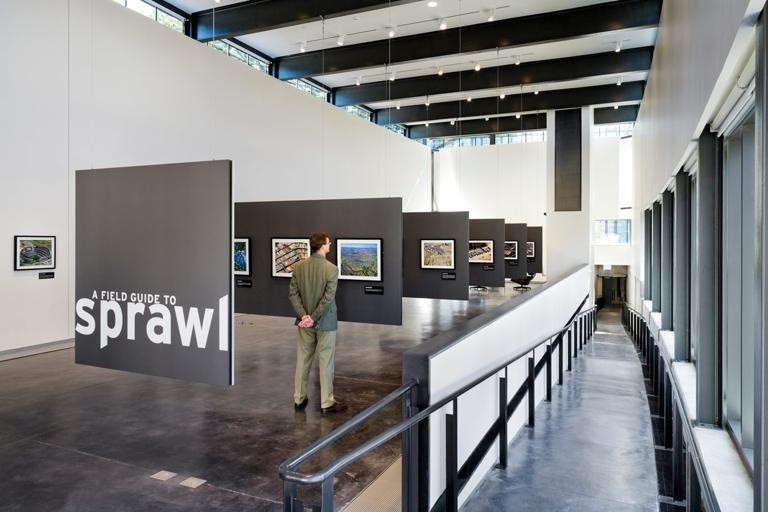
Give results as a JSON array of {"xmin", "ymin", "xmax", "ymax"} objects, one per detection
[{"xmin": 510, "ymin": 272, "xmax": 537, "ymax": 292}]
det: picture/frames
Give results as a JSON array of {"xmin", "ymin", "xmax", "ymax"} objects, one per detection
[
  {"xmin": 271, "ymin": 237, "xmax": 312, "ymax": 278},
  {"xmin": 234, "ymin": 237, "xmax": 251, "ymax": 276},
  {"xmin": 336, "ymin": 236, "xmax": 384, "ymax": 282},
  {"xmin": 504, "ymin": 241, "xmax": 519, "ymax": 260},
  {"xmin": 14, "ymin": 236, "xmax": 57, "ymax": 271},
  {"xmin": 419, "ymin": 238, "xmax": 457, "ymax": 271},
  {"xmin": 526, "ymin": 242, "xmax": 535, "ymax": 258},
  {"xmin": 468, "ymin": 239, "xmax": 494, "ymax": 264}
]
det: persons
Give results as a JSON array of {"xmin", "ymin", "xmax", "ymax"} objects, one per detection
[{"xmin": 289, "ymin": 231, "xmax": 346, "ymax": 416}]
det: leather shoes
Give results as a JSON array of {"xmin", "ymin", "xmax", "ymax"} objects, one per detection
[
  {"xmin": 321, "ymin": 402, "xmax": 346, "ymax": 413},
  {"xmin": 295, "ymin": 398, "xmax": 308, "ymax": 408}
]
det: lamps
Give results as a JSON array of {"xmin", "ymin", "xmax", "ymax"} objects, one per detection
[
  {"xmin": 439, "ymin": 17, "xmax": 448, "ymax": 31},
  {"xmin": 484, "ymin": 7, "xmax": 496, "ymax": 22},
  {"xmin": 386, "ymin": 27, "xmax": 396, "ymax": 39},
  {"xmin": 346, "ymin": 55, "xmax": 622, "ymax": 128},
  {"xmin": 336, "ymin": 35, "xmax": 344, "ymax": 46},
  {"xmin": 298, "ymin": 43, "xmax": 307, "ymax": 54},
  {"xmin": 612, "ymin": 39, "xmax": 621, "ymax": 54}
]
